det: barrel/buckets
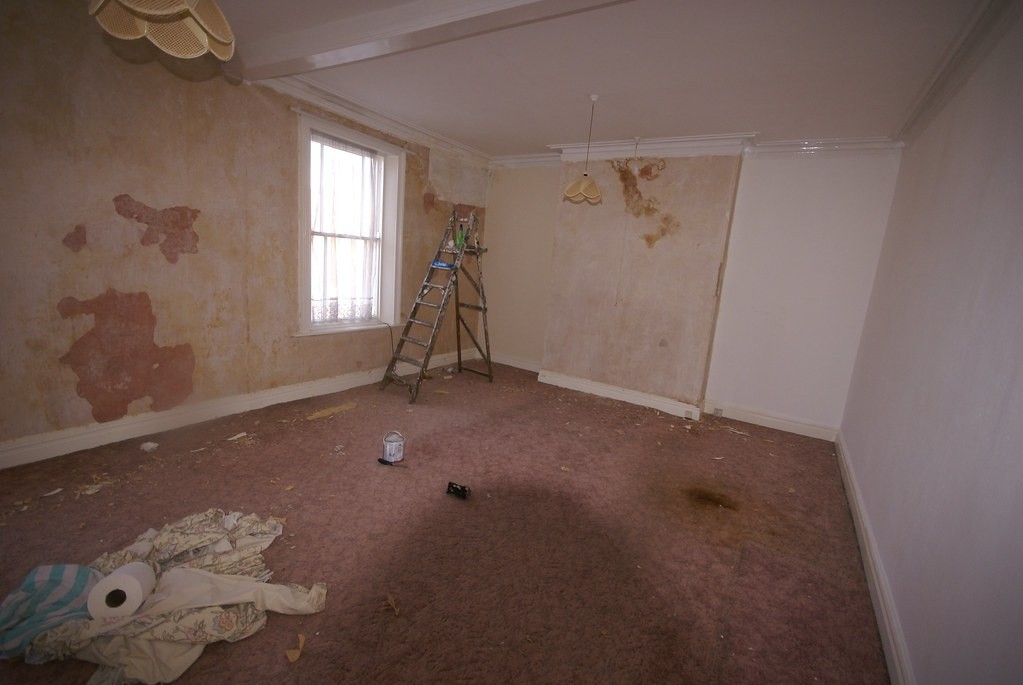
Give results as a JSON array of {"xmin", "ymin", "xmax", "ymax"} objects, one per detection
[{"xmin": 383, "ymin": 431, "xmax": 404, "ymax": 462}]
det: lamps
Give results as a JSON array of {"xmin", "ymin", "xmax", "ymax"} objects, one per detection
[
  {"xmin": 88, "ymin": 0, "xmax": 236, "ymax": 62},
  {"xmin": 563, "ymin": 94, "xmax": 604, "ymax": 205}
]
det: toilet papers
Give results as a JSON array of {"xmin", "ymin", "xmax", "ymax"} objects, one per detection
[{"xmin": 86, "ymin": 561, "xmax": 156, "ymax": 620}]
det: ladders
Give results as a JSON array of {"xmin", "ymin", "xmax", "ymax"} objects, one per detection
[{"xmin": 380, "ymin": 211, "xmax": 495, "ymax": 404}]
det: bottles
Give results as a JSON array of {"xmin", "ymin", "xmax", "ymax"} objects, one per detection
[{"xmin": 457, "ymin": 224, "xmax": 463, "ymax": 249}]
